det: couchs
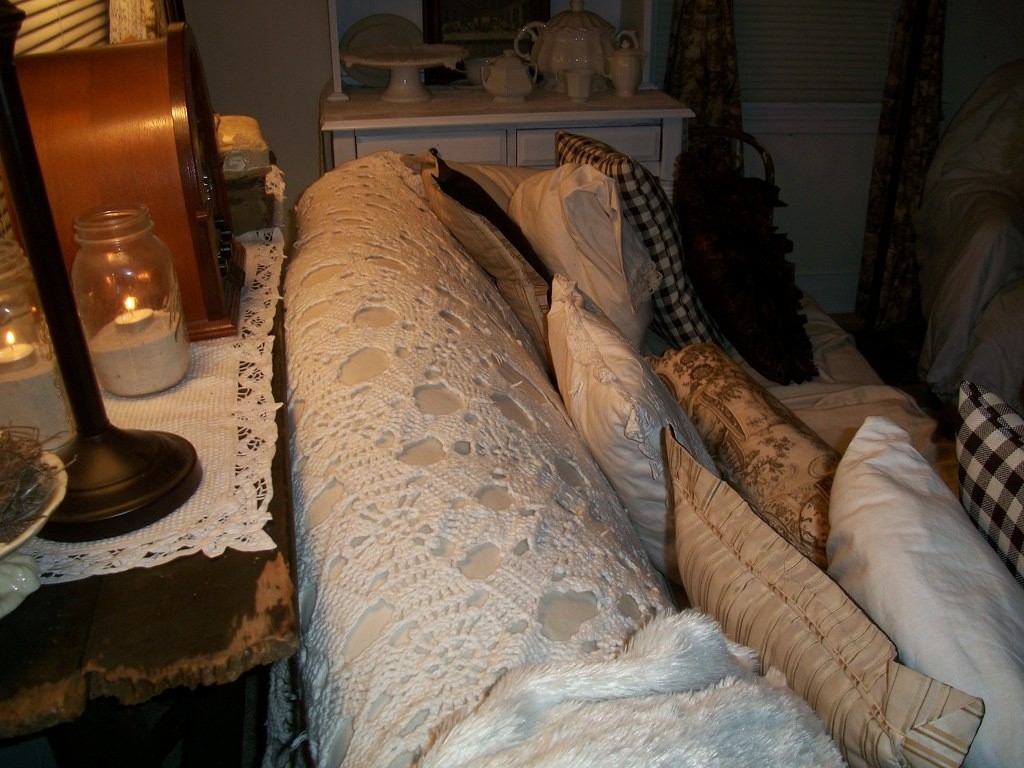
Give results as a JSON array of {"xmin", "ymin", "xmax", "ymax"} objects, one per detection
[{"xmin": 277, "ymin": 151, "xmax": 954, "ymax": 768}]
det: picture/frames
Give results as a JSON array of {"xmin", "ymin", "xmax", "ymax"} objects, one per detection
[{"xmin": 421, "ymin": 0, "xmax": 552, "ymax": 87}]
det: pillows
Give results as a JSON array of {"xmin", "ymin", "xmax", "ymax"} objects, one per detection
[
  {"xmin": 400, "ymin": 147, "xmax": 556, "ymax": 366},
  {"xmin": 656, "ymin": 340, "xmax": 847, "ymax": 565},
  {"xmin": 510, "ymin": 165, "xmax": 662, "ymax": 351},
  {"xmin": 672, "ymin": 128, "xmax": 822, "ymax": 381},
  {"xmin": 828, "ymin": 417, "xmax": 1024, "ymax": 768},
  {"xmin": 554, "ymin": 132, "xmax": 732, "ymax": 356},
  {"xmin": 545, "ymin": 272, "xmax": 722, "ymax": 587},
  {"xmin": 662, "ymin": 426, "xmax": 986, "ymax": 766},
  {"xmin": 959, "ymin": 383, "xmax": 1024, "ymax": 572}
]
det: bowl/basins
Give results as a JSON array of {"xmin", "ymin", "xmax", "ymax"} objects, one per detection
[
  {"xmin": 0, "ymin": 449, "xmax": 69, "ymax": 619},
  {"xmin": 340, "ymin": 44, "xmax": 468, "ymax": 104}
]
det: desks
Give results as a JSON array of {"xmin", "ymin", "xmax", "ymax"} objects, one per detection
[{"xmin": 0, "ymin": 163, "xmax": 297, "ymax": 767}]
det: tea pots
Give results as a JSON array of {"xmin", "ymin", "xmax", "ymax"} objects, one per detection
[{"xmin": 514, "ymin": 0, "xmax": 640, "ymax": 93}]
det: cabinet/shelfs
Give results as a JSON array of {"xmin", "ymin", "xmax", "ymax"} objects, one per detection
[{"xmin": 321, "ymin": 83, "xmax": 699, "ymax": 224}]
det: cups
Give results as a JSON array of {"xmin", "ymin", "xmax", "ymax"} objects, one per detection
[
  {"xmin": 480, "ymin": 50, "xmax": 538, "ymax": 104},
  {"xmin": 598, "ymin": 41, "xmax": 643, "ymax": 98},
  {"xmin": 558, "ymin": 68, "xmax": 596, "ymax": 105}
]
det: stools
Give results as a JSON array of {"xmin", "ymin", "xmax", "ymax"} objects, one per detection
[{"xmin": 215, "ymin": 113, "xmax": 293, "ymax": 242}]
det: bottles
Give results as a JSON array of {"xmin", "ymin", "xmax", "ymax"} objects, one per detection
[
  {"xmin": 69, "ymin": 200, "xmax": 193, "ymax": 397},
  {"xmin": 0, "ymin": 238, "xmax": 77, "ymax": 454}
]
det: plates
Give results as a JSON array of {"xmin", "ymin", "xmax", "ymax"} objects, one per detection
[{"xmin": 338, "ymin": 13, "xmax": 424, "ymax": 89}]
